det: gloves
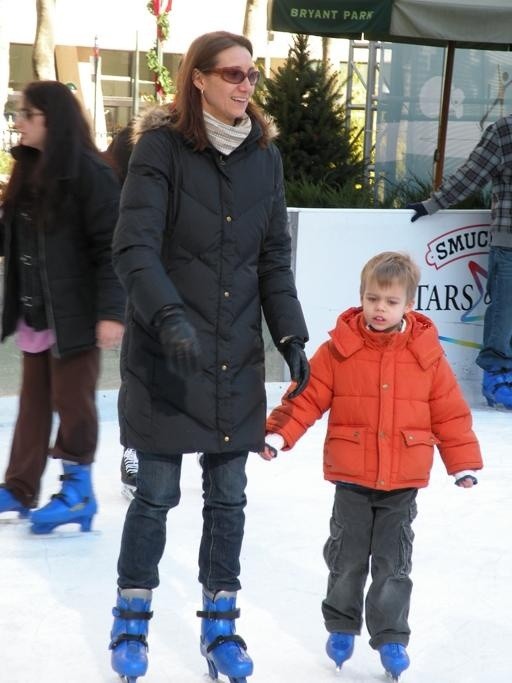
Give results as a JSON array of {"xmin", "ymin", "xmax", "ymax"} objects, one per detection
[
  {"xmin": 153, "ymin": 306, "xmax": 201, "ymax": 380},
  {"xmin": 282, "ymin": 337, "xmax": 310, "ymax": 399},
  {"xmin": 406, "ymin": 202, "xmax": 429, "ymax": 222}
]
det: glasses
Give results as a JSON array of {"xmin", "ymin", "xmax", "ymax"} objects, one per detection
[
  {"xmin": 205, "ymin": 68, "xmax": 260, "ymax": 86},
  {"xmin": 18, "ymin": 109, "xmax": 44, "ymax": 121}
]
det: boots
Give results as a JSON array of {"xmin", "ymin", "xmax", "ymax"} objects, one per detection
[
  {"xmin": 111, "ymin": 587, "xmax": 152, "ymax": 676},
  {"xmin": 30, "ymin": 462, "xmax": 95, "ymax": 524},
  {"xmin": 200, "ymin": 586, "xmax": 252, "ymax": 678},
  {"xmin": 0, "ymin": 485, "xmax": 29, "ymax": 520}
]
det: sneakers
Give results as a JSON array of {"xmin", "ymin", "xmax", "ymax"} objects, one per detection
[
  {"xmin": 380, "ymin": 644, "xmax": 409, "ymax": 674},
  {"xmin": 121, "ymin": 447, "xmax": 140, "ymax": 486},
  {"xmin": 327, "ymin": 631, "xmax": 354, "ymax": 662},
  {"xmin": 482, "ymin": 370, "xmax": 512, "ymax": 409}
]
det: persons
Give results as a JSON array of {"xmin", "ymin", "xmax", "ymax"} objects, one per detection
[
  {"xmin": 108, "ymin": 30, "xmax": 312, "ymax": 683},
  {"xmin": 408, "ymin": 116, "xmax": 512, "ymax": 411},
  {"xmin": 258, "ymin": 251, "xmax": 484, "ymax": 682},
  {"xmin": 1, "ymin": 80, "xmax": 128, "ymax": 534},
  {"xmin": 107, "ymin": 120, "xmax": 139, "ymax": 500}
]
